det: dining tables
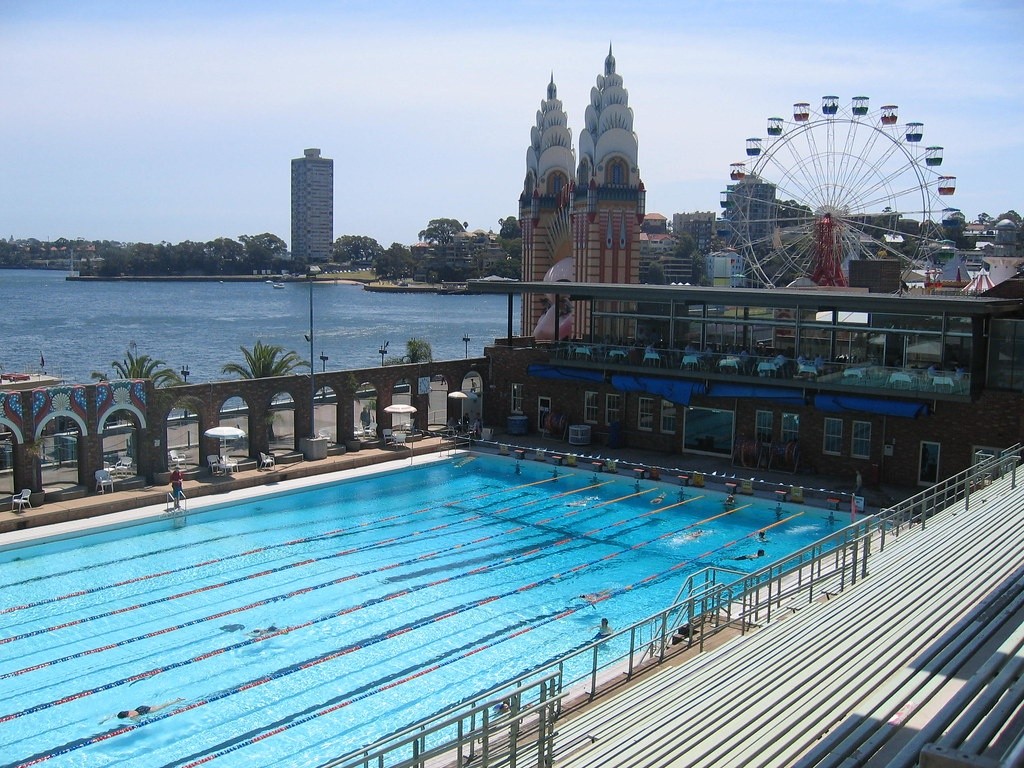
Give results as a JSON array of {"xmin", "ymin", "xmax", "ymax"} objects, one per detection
[
  {"xmin": 683, "ymin": 354, "xmax": 705, "ymax": 371},
  {"xmin": 933, "ymin": 374, "xmax": 962, "ymax": 393},
  {"xmin": 844, "ymin": 365, "xmax": 866, "ymax": 385},
  {"xmin": 609, "ymin": 348, "xmax": 626, "ymax": 362},
  {"xmin": 644, "ymin": 351, "xmax": 663, "ymax": 366},
  {"xmin": 892, "ymin": 371, "xmax": 917, "ymax": 390},
  {"xmin": 575, "ymin": 347, "xmax": 591, "ymax": 359},
  {"xmin": 799, "ymin": 362, "xmax": 819, "ymax": 380},
  {"xmin": 719, "ymin": 356, "xmax": 741, "ymax": 372},
  {"xmin": 758, "ymin": 359, "xmax": 778, "ymax": 377}
]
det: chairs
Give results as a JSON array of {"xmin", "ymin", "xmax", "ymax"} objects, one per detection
[
  {"xmin": 170, "ymin": 449, "xmax": 187, "ymax": 469},
  {"xmin": 95, "ymin": 469, "xmax": 113, "ymax": 493},
  {"xmin": 11, "ymin": 488, "xmax": 33, "ymax": 512},
  {"xmin": 207, "ymin": 453, "xmax": 238, "ymax": 475},
  {"xmin": 104, "ymin": 460, "xmax": 117, "ymax": 478},
  {"xmin": 558, "ymin": 336, "xmax": 972, "ymax": 395},
  {"xmin": 115, "ymin": 456, "xmax": 133, "ymax": 476},
  {"xmin": 383, "ymin": 428, "xmax": 396, "ymax": 446},
  {"xmin": 259, "ymin": 451, "xmax": 276, "ymax": 470},
  {"xmin": 392, "ymin": 432, "xmax": 407, "ymax": 449},
  {"xmin": 317, "ymin": 422, "xmax": 378, "ymax": 445}
]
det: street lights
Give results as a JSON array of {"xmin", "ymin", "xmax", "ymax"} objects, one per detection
[
  {"xmin": 181, "ymin": 371, "xmax": 189, "ymax": 420},
  {"xmin": 320, "ymin": 356, "xmax": 328, "ymax": 397},
  {"xmin": 378, "ymin": 350, "xmax": 387, "ymax": 367},
  {"xmin": 463, "ymin": 338, "xmax": 470, "ymax": 358},
  {"xmin": 308, "ymin": 266, "xmax": 320, "ymax": 438}
]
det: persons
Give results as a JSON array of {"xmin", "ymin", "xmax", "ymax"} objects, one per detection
[
  {"xmin": 773, "ymin": 352, "xmax": 786, "ymax": 368},
  {"xmin": 170, "ymin": 467, "xmax": 183, "ymax": 508},
  {"xmin": 738, "ymin": 550, "xmax": 765, "ymax": 560},
  {"xmin": 470, "ymin": 379, "xmax": 477, "ymax": 392},
  {"xmin": 705, "ymin": 344, "xmax": 755, "ymax": 358},
  {"xmin": 440, "ymin": 376, "xmax": 447, "ymax": 385},
  {"xmin": 360, "ymin": 407, "xmax": 370, "ymax": 434},
  {"xmin": 756, "ymin": 532, "xmax": 767, "ymax": 541},
  {"xmin": 921, "ymin": 443, "xmax": 929, "ymax": 475},
  {"xmin": 854, "ymin": 470, "xmax": 862, "ymax": 495},
  {"xmin": 500, "ymin": 699, "xmax": 510, "ymax": 712},
  {"xmin": 561, "ymin": 335, "xmax": 578, "ymax": 345},
  {"xmin": 244, "ymin": 622, "xmax": 288, "ymax": 637},
  {"xmin": 645, "ymin": 332, "xmax": 657, "ymax": 353},
  {"xmin": 685, "ymin": 342, "xmax": 697, "ymax": 356},
  {"xmin": 448, "ymin": 413, "xmax": 483, "ymax": 434},
  {"xmin": 927, "ymin": 365, "xmax": 963, "ymax": 383},
  {"xmin": 723, "ymin": 495, "xmax": 735, "ymax": 505},
  {"xmin": 590, "ymin": 617, "xmax": 613, "ymax": 636},
  {"xmin": 797, "ymin": 352, "xmax": 823, "ymax": 370},
  {"xmin": 99, "ymin": 697, "xmax": 185, "ymax": 724}
]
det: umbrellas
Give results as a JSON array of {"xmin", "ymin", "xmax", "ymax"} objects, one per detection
[
  {"xmin": 449, "ymin": 391, "xmax": 477, "ymax": 424},
  {"xmin": 383, "ymin": 404, "xmax": 417, "ymax": 433},
  {"xmin": 204, "ymin": 427, "xmax": 246, "ymax": 475}
]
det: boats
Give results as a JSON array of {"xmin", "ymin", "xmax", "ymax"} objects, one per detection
[
  {"xmin": 273, "ymin": 282, "xmax": 285, "ymax": 289},
  {"xmin": 265, "ymin": 280, "xmax": 273, "ymax": 284}
]
